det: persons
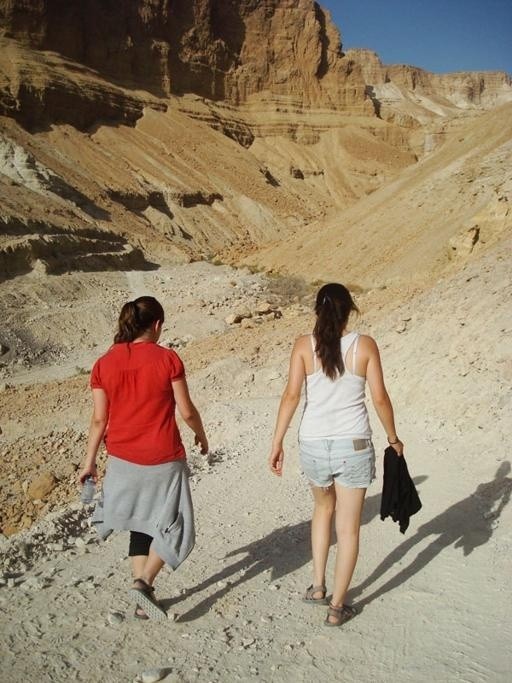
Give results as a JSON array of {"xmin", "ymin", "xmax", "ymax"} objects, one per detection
[
  {"xmin": 269, "ymin": 282, "xmax": 403, "ymax": 629},
  {"xmin": 76, "ymin": 296, "xmax": 210, "ymax": 625}
]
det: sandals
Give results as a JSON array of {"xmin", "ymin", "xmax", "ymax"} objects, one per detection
[
  {"xmin": 301, "ymin": 584, "xmax": 358, "ymax": 627},
  {"xmin": 129, "ymin": 578, "xmax": 168, "ymax": 625}
]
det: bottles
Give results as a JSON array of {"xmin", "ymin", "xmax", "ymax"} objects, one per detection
[{"xmin": 80, "ymin": 464, "xmax": 100, "ymax": 503}]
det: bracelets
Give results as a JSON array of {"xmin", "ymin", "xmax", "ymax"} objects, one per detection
[{"xmin": 388, "ymin": 435, "xmax": 400, "ymax": 446}]
